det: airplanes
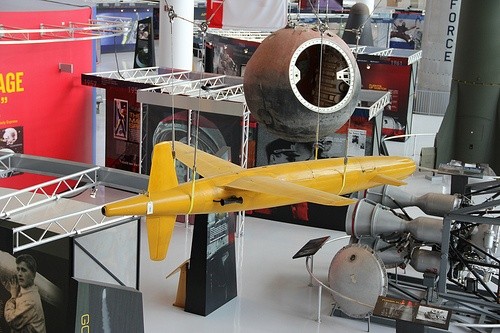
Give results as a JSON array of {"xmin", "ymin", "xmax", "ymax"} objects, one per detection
[{"xmin": 104, "ymin": 141, "xmax": 417, "ymax": 261}]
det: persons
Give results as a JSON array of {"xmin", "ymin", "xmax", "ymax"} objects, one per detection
[
  {"xmin": 266, "ymin": 137, "xmax": 300, "ymax": 165},
  {"xmin": 115, "ymin": 105, "xmax": 126, "ymax": 137},
  {"xmin": 4, "ymin": 253, "xmax": 47, "ymax": 332}
]
